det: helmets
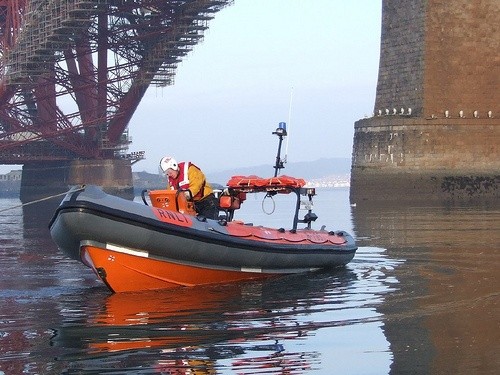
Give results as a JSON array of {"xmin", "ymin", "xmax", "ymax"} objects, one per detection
[{"xmin": 160, "ymin": 156, "xmax": 179, "ymax": 171}]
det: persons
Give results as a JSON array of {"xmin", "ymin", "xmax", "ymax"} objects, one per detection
[{"xmin": 160, "ymin": 156, "xmax": 219, "ymax": 221}]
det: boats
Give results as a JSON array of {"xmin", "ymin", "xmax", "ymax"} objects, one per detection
[
  {"xmin": 48, "ymin": 122, "xmax": 359, "ymax": 294},
  {"xmin": 87, "ymin": 291, "xmax": 308, "ymax": 354}
]
